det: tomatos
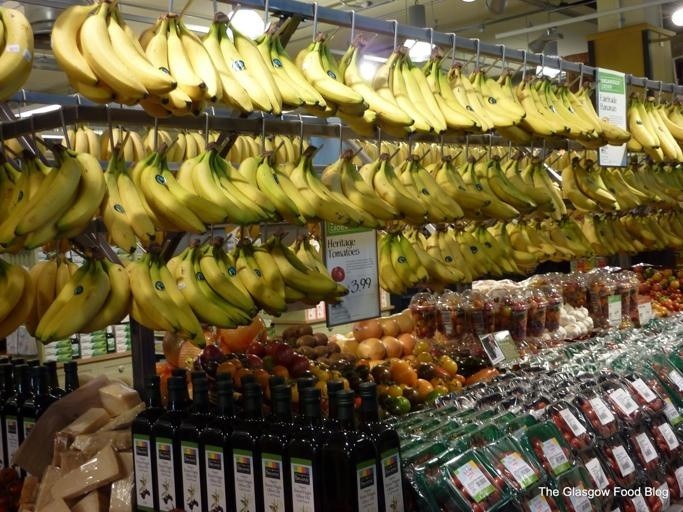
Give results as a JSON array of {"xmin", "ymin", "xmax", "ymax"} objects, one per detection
[{"xmin": 452, "ymin": 361, "xmax": 683, "ymax": 512}]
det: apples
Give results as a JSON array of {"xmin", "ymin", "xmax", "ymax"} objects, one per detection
[{"xmin": 411, "ymin": 378, "xmax": 434, "ymax": 404}]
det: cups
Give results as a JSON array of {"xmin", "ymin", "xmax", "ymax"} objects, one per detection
[
  {"xmin": 409, "ymin": 299, "xmax": 562, "ymax": 341},
  {"xmin": 563, "ymin": 283, "xmax": 639, "ymax": 316}
]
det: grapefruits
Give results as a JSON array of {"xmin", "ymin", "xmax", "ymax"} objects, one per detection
[{"xmin": 157, "ymin": 309, "xmax": 267, "ymax": 409}]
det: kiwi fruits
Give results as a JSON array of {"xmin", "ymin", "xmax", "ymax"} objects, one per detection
[{"xmin": 271, "ymin": 325, "xmax": 356, "ymax": 366}]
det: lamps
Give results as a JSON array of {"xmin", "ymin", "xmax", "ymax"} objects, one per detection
[
  {"xmin": 485, "ymin": 0, "xmax": 505, "ymax": 14},
  {"xmin": 528, "ymin": 14, "xmax": 557, "ymax": 53},
  {"xmin": 403, "ymin": 0, "xmax": 437, "ymax": 62},
  {"xmin": 225, "ymin": 3, "xmax": 265, "ymax": 41}
]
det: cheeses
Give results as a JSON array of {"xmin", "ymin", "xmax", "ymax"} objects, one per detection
[{"xmin": 12, "ymin": 376, "xmax": 145, "ymax": 512}]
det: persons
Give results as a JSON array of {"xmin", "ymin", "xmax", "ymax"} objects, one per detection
[
  {"xmin": 631, "ymin": 206, "xmax": 676, "ymax": 270},
  {"xmin": 389, "ymin": 226, "xmax": 457, "ymax": 314}
]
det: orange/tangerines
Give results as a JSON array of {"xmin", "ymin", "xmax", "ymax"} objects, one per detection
[
  {"xmin": 425, "ymin": 390, "xmax": 445, "ymax": 403},
  {"xmin": 391, "ymin": 396, "xmax": 412, "ymax": 414}
]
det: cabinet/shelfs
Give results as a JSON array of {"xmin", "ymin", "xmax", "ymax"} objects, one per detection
[
  {"xmin": 270, "ymin": 304, "xmax": 395, "ymax": 337},
  {"xmin": 0, "ymin": 350, "xmax": 134, "ymax": 391}
]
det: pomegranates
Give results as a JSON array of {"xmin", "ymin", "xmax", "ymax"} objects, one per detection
[{"xmin": 342, "ymin": 308, "xmax": 417, "ymax": 371}]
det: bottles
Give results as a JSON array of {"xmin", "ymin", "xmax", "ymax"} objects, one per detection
[{"xmin": 131, "ymin": 368, "xmax": 405, "ymax": 511}]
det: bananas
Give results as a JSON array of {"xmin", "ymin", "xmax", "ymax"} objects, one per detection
[{"xmin": 0, "ymin": 0, "xmax": 683, "ymax": 349}]
